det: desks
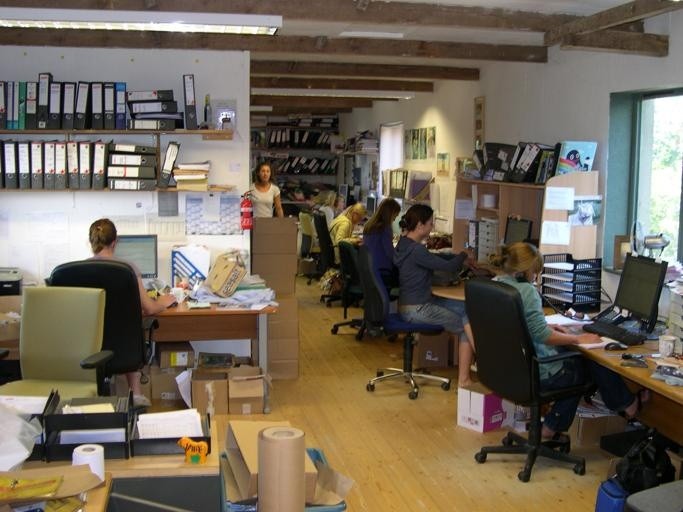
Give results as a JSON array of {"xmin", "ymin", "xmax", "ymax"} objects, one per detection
[
  {"xmin": 543, "ymin": 304, "xmax": 683, "ymax": 481},
  {"xmin": 143, "ymin": 301, "xmax": 278, "ymax": 413}
]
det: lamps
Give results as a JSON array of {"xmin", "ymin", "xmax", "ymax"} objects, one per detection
[
  {"xmin": 0, "ymin": 7, "xmax": 284, "ymax": 36},
  {"xmin": 251, "ymin": 87, "xmax": 416, "ymax": 99}
]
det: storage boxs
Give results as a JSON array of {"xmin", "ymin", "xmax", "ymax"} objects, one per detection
[
  {"xmin": 252, "ymin": 218, "xmax": 300, "ymax": 381},
  {"xmin": 222, "ymin": 458, "xmax": 258, "ymax": 505},
  {"xmin": 568, "ymin": 416, "xmax": 626, "ymax": 454},
  {"xmin": 221, "ymin": 419, "xmax": 318, "ymax": 503},
  {"xmin": 113, "ymin": 340, "xmax": 274, "ymax": 414}
]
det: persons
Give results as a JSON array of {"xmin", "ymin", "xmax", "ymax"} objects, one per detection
[
  {"xmin": 491, "ymin": 243, "xmax": 651, "ymax": 442},
  {"xmin": 308, "ymin": 191, "xmax": 345, "ymax": 246},
  {"xmin": 363, "ymin": 199, "xmax": 401, "ymax": 286},
  {"xmin": 250, "ymin": 162, "xmax": 284, "ymax": 217},
  {"xmin": 393, "ymin": 204, "xmax": 476, "ymax": 387},
  {"xmin": 328, "ymin": 202, "xmax": 367, "ymax": 264},
  {"xmin": 86, "ymin": 219, "xmax": 176, "ymax": 406}
]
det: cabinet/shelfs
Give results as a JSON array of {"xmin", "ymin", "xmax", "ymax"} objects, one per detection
[
  {"xmin": 452, "ymin": 171, "xmax": 599, "ymax": 267},
  {"xmin": 0, "ymin": 129, "xmax": 235, "ymax": 193},
  {"xmin": 250, "ymin": 126, "xmax": 345, "ymax": 205}
]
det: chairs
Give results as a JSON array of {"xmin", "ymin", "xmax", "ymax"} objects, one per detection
[
  {"xmin": 0, "ymin": 286, "xmax": 114, "ymax": 401},
  {"xmin": 45, "ymin": 259, "xmax": 159, "ymax": 414}
]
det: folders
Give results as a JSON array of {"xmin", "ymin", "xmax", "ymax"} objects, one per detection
[
  {"xmin": 0, "ymin": 73, "xmax": 198, "ymax": 191},
  {"xmin": 390, "ymin": 170, "xmax": 408, "ymax": 198},
  {"xmin": 268, "ymin": 118, "xmax": 339, "ymax": 174},
  {"xmin": 473, "ymin": 142, "xmax": 556, "ymax": 182}
]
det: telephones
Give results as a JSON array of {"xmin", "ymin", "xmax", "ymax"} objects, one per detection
[{"xmin": 515, "ymin": 272, "xmax": 529, "ymax": 282}]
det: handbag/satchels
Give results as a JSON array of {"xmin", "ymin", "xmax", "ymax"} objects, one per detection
[
  {"xmin": 318, "ymin": 266, "xmax": 345, "ymax": 296},
  {"xmin": 615, "ymin": 424, "xmax": 677, "ymax": 493},
  {"xmin": 203, "ymin": 246, "xmax": 249, "ymax": 299}
]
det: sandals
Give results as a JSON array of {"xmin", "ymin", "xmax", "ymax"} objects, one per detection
[
  {"xmin": 624, "ymin": 387, "xmax": 651, "ymax": 420},
  {"xmin": 541, "ymin": 430, "xmax": 571, "ymax": 444}
]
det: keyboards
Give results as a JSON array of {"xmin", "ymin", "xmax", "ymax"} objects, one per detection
[{"xmin": 582, "ymin": 319, "xmax": 648, "ymax": 346}]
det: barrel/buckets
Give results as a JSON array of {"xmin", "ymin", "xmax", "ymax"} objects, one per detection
[{"xmin": 596, "ymin": 478, "xmax": 624, "ymax": 510}]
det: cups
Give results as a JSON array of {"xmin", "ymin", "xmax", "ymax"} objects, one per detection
[
  {"xmin": 481, "ymin": 193, "xmax": 495, "ymax": 209},
  {"xmin": 657, "ymin": 335, "xmax": 676, "ymax": 358},
  {"xmin": 221, "ymin": 117, "xmax": 232, "ymax": 130},
  {"xmin": 175, "ymin": 277, "xmax": 189, "ymax": 293}
]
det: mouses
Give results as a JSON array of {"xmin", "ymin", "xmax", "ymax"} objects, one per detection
[
  {"xmin": 168, "ymin": 301, "xmax": 178, "ymax": 308},
  {"xmin": 606, "ymin": 339, "xmax": 622, "ymax": 351}
]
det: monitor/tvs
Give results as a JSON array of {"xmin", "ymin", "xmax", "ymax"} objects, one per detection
[
  {"xmin": 502, "ymin": 217, "xmax": 533, "ymax": 250},
  {"xmin": 366, "ymin": 195, "xmax": 377, "ymax": 217},
  {"xmin": 394, "ymin": 197, "xmax": 403, "ymax": 213},
  {"xmin": 467, "ymin": 220, "xmax": 479, "ymax": 252},
  {"xmin": 109, "ymin": 233, "xmax": 160, "ymax": 281},
  {"xmin": 337, "ymin": 183, "xmax": 350, "ymax": 208},
  {"xmin": 353, "ymin": 185, "xmax": 361, "ymax": 201}
]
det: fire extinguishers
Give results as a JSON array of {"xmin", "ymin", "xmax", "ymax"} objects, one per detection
[{"xmin": 240, "ymin": 190, "xmax": 253, "ymax": 230}]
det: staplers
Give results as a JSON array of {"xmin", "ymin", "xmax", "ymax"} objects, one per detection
[{"xmin": 621, "ymin": 353, "xmax": 649, "ymax": 368}]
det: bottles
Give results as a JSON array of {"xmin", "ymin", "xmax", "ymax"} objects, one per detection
[{"xmin": 203, "ymin": 93, "xmax": 213, "ymax": 129}]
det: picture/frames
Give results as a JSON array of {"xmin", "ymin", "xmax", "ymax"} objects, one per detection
[{"xmin": 474, "ymin": 95, "xmax": 485, "ymax": 150}]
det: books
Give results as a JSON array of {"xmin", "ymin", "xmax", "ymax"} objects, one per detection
[{"xmin": 172, "ymin": 160, "xmax": 210, "ymax": 191}]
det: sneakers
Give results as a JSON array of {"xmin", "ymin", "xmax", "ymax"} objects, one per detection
[{"xmin": 132, "ymin": 393, "xmax": 152, "ymax": 409}]
